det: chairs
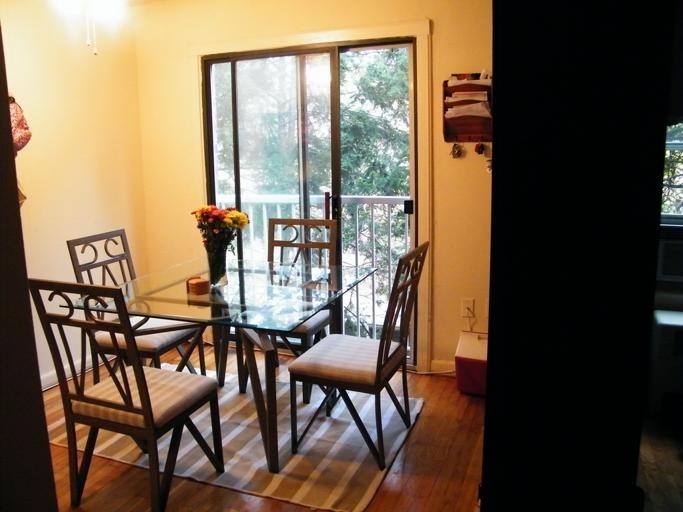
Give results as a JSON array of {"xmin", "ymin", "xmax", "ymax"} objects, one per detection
[
  {"xmin": 28, "ymin": 277, "xmax": 224, "ymax": 511},
  {"xmin": 235, "ymin": 219, "xmax": 342, "ymax": 394},
  {"xmin": 66, "ymin": 228, "xmax": 206, "ymax": 385},
  {"xmin": 289, "ymin": 241, "xmax": 431, "ymax": 471}
]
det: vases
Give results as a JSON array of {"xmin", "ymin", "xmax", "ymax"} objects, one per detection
[{"xmin": 206, "ymin": 249, "xmax": 227, "ymax": 295}]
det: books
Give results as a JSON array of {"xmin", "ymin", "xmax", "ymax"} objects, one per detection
[{"xmin": 445, "ymin": 76, "xmax": 492, "ymax": 119}]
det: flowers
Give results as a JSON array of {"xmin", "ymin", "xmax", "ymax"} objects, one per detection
[{"xmin": 191, "ymin": 202, "xmax": 249, "ymax": 257}]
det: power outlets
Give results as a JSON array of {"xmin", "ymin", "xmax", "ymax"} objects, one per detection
[{"xmin": 460, "ymin": 299, "xmax": 474, "ymax": 318}]
